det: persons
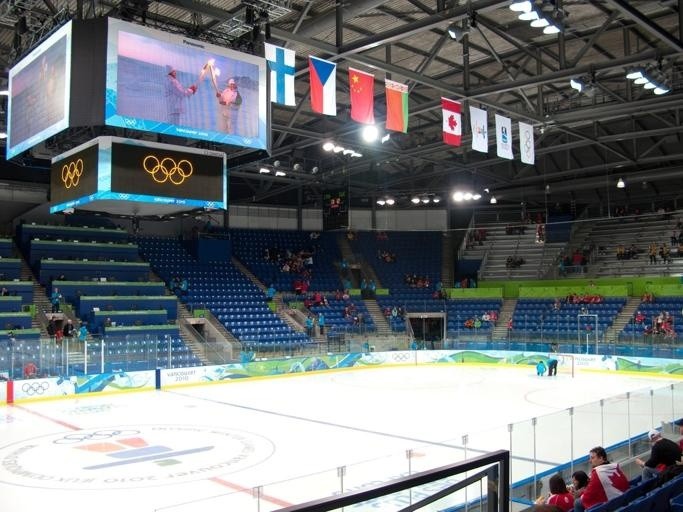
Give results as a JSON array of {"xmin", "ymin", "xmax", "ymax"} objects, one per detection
[
  {"xmin": 564, "ymin": 469, "xmax": 590, "ymax": 498},
  {"xmin": 632, "ymin": 429, "xmax": 681, "ymax": 483},
  {"xmin": 49, "ymin": 287, "xmax": 63, "ymax": 314},
  {"xmin": 532, "ymin": 474, "xmax": 574, "ymax": 511},
  {"xmin": 0, "ymin": 286, "xmax": 10, "ymax": 297},
  {"xmin": 535, "ymin": 360, "xmax": 546, "ymax": 377},
  {"xmin": 260, "ymin": 212, "xmax": 682, "ymax": 353},
  {"xmin": 22, "ymin": 359, "xmax": 38, "ymax": 378},
  {"xmin": 47, "ymin": 316, "xmax": 58, "ymax": 335},
  {"xmin": 60, "ymin": 319, "xmax": 74, "ymax": 338},
  {"xmin": 71, "ymin": 317, "xmax": 89, "ymax": 343},
  {"xmin": 214, "ymin": 79, "xmax": 242, "ymax": 130},
  {"xmin": 672, "ymin": 417, "xmax": 683, "ymax": 465},
  {"xmin": 545, "ymin": 358, "xmax": 558, "ymax": 376},
  {"xmin": 171, "ymin": 276, "xmax": 183, "ymax": 292},
  {"xmin": 573, "ymin": 446, "xmax": 630, "ymax": 511},
  {"xmin": 163, "ymin": 63, "xmax": 199, "ymax": 128}
]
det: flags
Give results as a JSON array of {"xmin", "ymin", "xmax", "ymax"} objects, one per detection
[
  {"xmin": 438, "ymin": 96, "xmax": 462, "ymax": 147},
  {"xmin": 383, "ymin": 79, "xmax": 408, "ymax": 135},
  {"xmin": 519, "ymin": 120, "xmax": 536, "ymax": 166},
  {"xmin": 304, "ymin": 55, "xmax": 340, "ymax": 117},
  {"xmin": 469, "ymin": 107, "xmax": 490, "ymax": 155},
  {"xmin": 347, "ymin": 68, "xmax": 377, "ymax": 128},
  {"xmin": 262, "ymin": 42, "xmax": 298, "ymax": 109},
  {"xmin": 494, "ymin": 113, "xmax": 515, "ymax": 162}
]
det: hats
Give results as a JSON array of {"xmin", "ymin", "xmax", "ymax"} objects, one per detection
[{"xmin": 647, "ymin": 428, "xmax": 660, "ymax": 442}]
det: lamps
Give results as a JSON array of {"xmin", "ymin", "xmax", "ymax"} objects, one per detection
[
  {"xmin": 260, "ymin": 142, "xmax": 498, "ymax": 205},
  {"xmin": 616, "ymin": 177, "xmax": 626, "ymax": 188},
  {"xmin": 509, "ymin": 0, "xmax": 563, "ymax": 35},
  {"xmin": 625, "ymin": 58, "xmax": 671, "ymax": 96}
]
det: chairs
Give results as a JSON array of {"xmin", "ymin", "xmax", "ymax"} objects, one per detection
[
  {"xmin": 566, "ymin": 464, "xmax": 683, "ymax": 512},
  {"xmin": 0, "ymin": 213, "xmax": 683, "ymax": 383}
]
